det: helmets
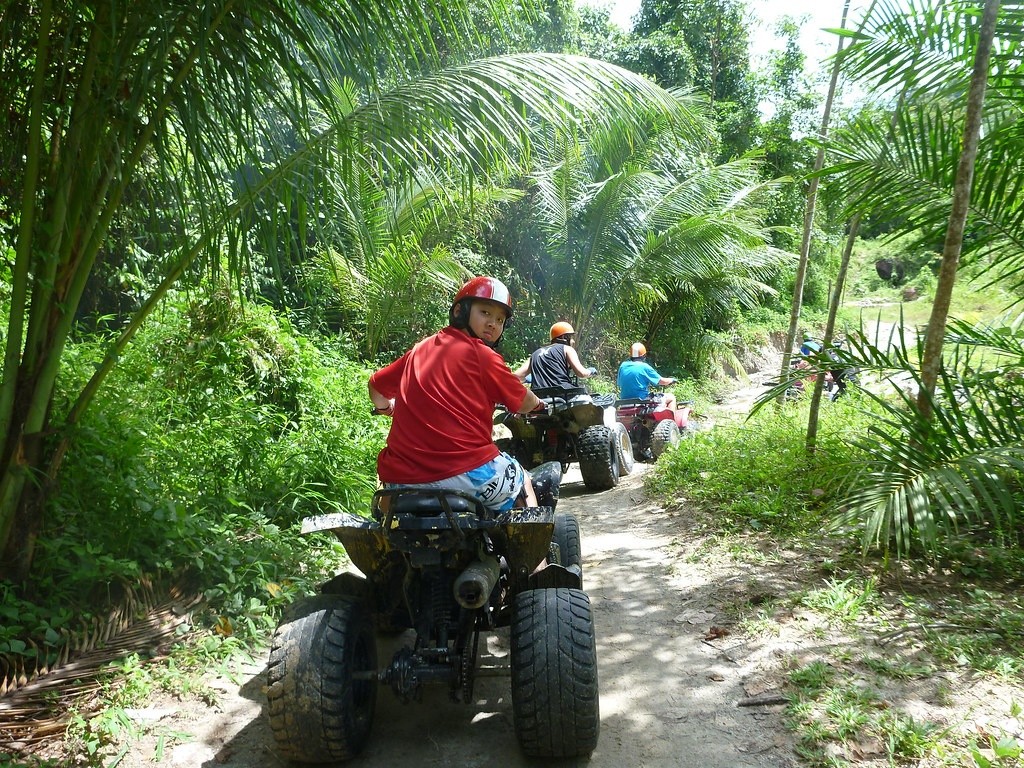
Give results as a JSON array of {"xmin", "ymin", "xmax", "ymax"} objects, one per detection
[
  {"xmin": 629, "ymin": 343, "xmax": 646, "ymax": 359},
  {"xmin": 549, "ymin": 322, "xmax": 578, "ymax": 343},
  {"xmin": 803, "ymin": 332, "xmax": 812, "ymax": 340},
  {"xmin": 452, "ymin": 276, "xmax": 514, "ymax": 319}
]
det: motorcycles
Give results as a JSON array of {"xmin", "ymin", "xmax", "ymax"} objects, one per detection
[
  {"xmin": 267, "ymin": 404, "xmax": 602, "ymax": 768},
  {"xmin": 489, "ymin": 368, "xmax": 635, "ymax": 493},
  {"xmin": 606, "ymin": 376, "xmax": 696, "ymax": 465},
  {"xmin": 782, "ymin": 350, "xmax": 862, "ymax": 410}
]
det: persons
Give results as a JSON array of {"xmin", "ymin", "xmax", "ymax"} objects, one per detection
[
  {"xmin": 513, "ymin": 321, "xmax": 596, "ymax": 389},
  {"xmin": 367, "ymin": 276, "xmax": 548, "ymax": 574},
  {"xmin": 800, "ymin": 333, "xmax": 820, "ymax": 355},
  {"xmin": 615, "ymin": 342, "xmax": 676, "ymax": 417}
]
